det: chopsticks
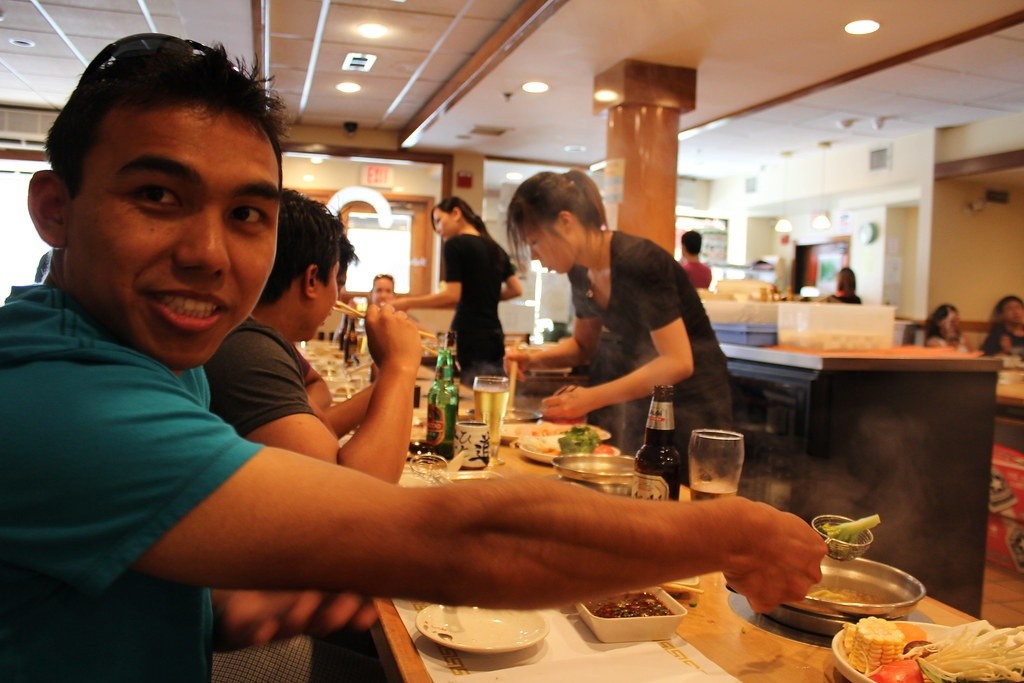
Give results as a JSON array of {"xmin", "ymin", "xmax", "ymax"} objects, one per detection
[{"xmin": 332, "ymin": 300, "xmax": 439, "ymax": 357}]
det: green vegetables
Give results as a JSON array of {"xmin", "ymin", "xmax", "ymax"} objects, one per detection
[{"xmin": 557, "ymin": 426, "xmax": 601, "ymax": 455}]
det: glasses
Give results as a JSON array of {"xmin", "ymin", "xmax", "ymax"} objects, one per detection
[
  {"xmin": 79, "ymin": 33, "xmax": 260, "ymax": 99},
  {"xmin": 376, "ymin": 274, "xmax": 393, "ymax": 281}
]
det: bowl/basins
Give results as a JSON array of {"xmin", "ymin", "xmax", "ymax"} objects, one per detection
[{"xmin": 574, "ymin": 584, "xmax": 689, "ymax": 643}]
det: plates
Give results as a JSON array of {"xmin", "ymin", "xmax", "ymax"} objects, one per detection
[
  {"xmin": 672, "ymin": 575, "xmax": 700, "ymax": 587},
  {"xmin": 500, "ymin": 424, "xmax": 611, "ymax": 443},
  {"xmin": 767, "ymin": 605, "xmax": 852, "ymax": 636},
  {"xmin": 830, "ymin": 621, "xmax": 950, "ymax": 683},
  {"xmin": 415, "ymin": 604, "xmax": 550, "ymax": 654},
  {"xmin": 519, "ymin": 435, "xmax": 567, "ymax": 464},
  {"xmin": 725, "ymin": 557, "xmax": 928, "ymax": 618},
  {"xmin": 467, "ymin": 408, "xmax": 543, "ymax": 424},
  {"xmin": 299, "ymin": 340, "xmax": 370, "ymax": 403},
  {"xmin": 551, "ymin": 452, "xmax": 636, "ymax": 484}
]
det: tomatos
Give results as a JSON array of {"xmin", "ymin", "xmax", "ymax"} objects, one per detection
[
  {"xmin": 593, "ymin": 445, "xmax": 615, "ymax": 455},
  {"xmin": 868, "ymin": 660, "xmax": 925, "ymax": 683}
]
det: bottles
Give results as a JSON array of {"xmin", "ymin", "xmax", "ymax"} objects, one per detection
[
  {"xmin": 442, "ymin": 331, "xmax": 462, "ymax": 387},
  {"xmin": 425, "ymin": 349, "xmax": 459, "ymax": 461},
  {"xmin": 759, "ymin": 284, "xmax": 795, "ymax": 302},
  {"xmin": 338, "ymin": 313, "xmax": 358, "ymax": 366},
  {"xmin": 631, "ymin": 383, "xmax": 683, "ymax": 502}
]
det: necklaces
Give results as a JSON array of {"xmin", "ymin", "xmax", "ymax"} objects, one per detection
[{"xmin": 586, "ymin": 232, "xmax": 604, "ymax": 297}]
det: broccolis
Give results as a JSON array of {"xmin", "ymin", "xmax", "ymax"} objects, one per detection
[{"xmin": 817, "ymin": 514, "xmax": 880, "ymax": 545}]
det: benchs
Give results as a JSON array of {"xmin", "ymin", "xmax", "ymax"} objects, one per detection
[{"xmin": 915, "ymin": 322, "xmax": 991, "ymax": 350}]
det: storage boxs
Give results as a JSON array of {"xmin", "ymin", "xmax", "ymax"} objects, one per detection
[{"xmin": 776, "ymin": 302, "xmax": 896, "ymax": 347}]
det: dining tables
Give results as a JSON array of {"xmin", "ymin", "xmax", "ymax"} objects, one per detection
[{"xmin": 297, "ymin": 339, "xmax": 980, "ymax": 683}]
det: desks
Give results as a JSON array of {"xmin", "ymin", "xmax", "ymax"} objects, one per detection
[{"xmin": 995, "ymin": 369, "xmax": 1024, "ymax": 426}]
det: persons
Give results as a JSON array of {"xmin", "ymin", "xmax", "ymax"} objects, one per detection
[
  {"xmin": 979, "ymin": 294, "xmax": 1024, "ymax": 356},
  {"xmin": 385, "ymin": 196, "xmax": 522, "ymax": 383},
  {"xmin": 926, "ymin": 304, "xmax": 973, "ymax": 353},
  {"xmin": 310, "ymin": 232, "xmax": 360, "ymax": 344},
  {"xmin": 503, "ymin": 170, "xmax": 731, "ymax": 467},
  {"xmin": 820, "ymin": 267, "xmax": 860, "ymax": 303},
  {"xmin": 204, "ymin": 189, "xmax": 422, "ymax": 683},
  {"xmin": 0, "ymin": 32, "xmax": 828, "ymax": 683},
  {"xmin": 334, "ymin": 274, "xmax": 437, "ymax": 341},
  {"xmin": 681, "ymin": 231, "xmax": 712, "ymax": 288}
]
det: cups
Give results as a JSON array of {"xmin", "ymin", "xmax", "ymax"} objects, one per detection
[
  {"xmin": 453, "ymin": 421, "xmax": 491, "ymax": 470},
  {"xmin": 687, "ymin": 429, "xmax": 745, "ymax": 502},
  {"xmin": 472, "ymin": 376, "xmax": 511, "ymax": 467}
]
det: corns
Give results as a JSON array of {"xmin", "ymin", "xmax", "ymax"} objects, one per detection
[{"xmin": 848, "ymin": 616, "xmax": 904, "ymax": 673}]
